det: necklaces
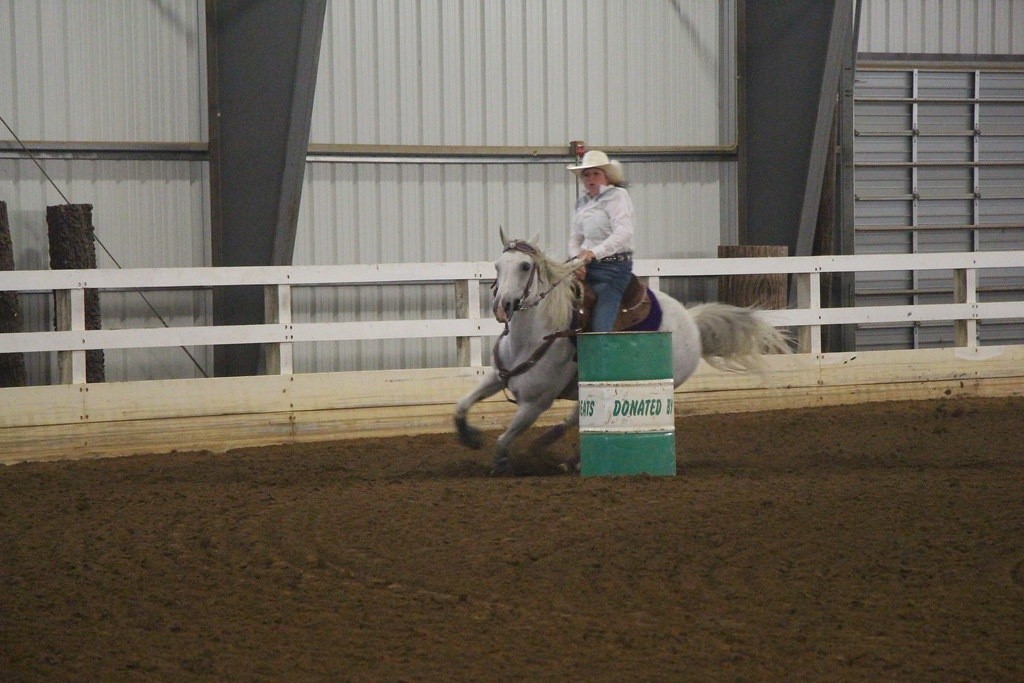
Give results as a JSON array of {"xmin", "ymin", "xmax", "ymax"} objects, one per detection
[{"xmin": 587, "ymin": 192, "xmax": 598, "ymax": 199}]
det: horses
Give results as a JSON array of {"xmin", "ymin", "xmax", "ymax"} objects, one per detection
[{"xmin": 453, "ymin": 225, "xmax": 802, "ymax": 467}]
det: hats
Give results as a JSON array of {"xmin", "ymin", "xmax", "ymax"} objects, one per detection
[{"xmin": 566, "ymin": 150, "xmax": 625, "ymax": 184}]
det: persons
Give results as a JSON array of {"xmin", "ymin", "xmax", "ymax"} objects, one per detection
[{"xmin": 564, "ymin": 150, "xmax": 635, "ymax": 331}]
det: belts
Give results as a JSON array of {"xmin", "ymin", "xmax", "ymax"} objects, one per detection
[{"xmin": 593, "ymin": 252, "xmax": 632, "ymax": 262}]
